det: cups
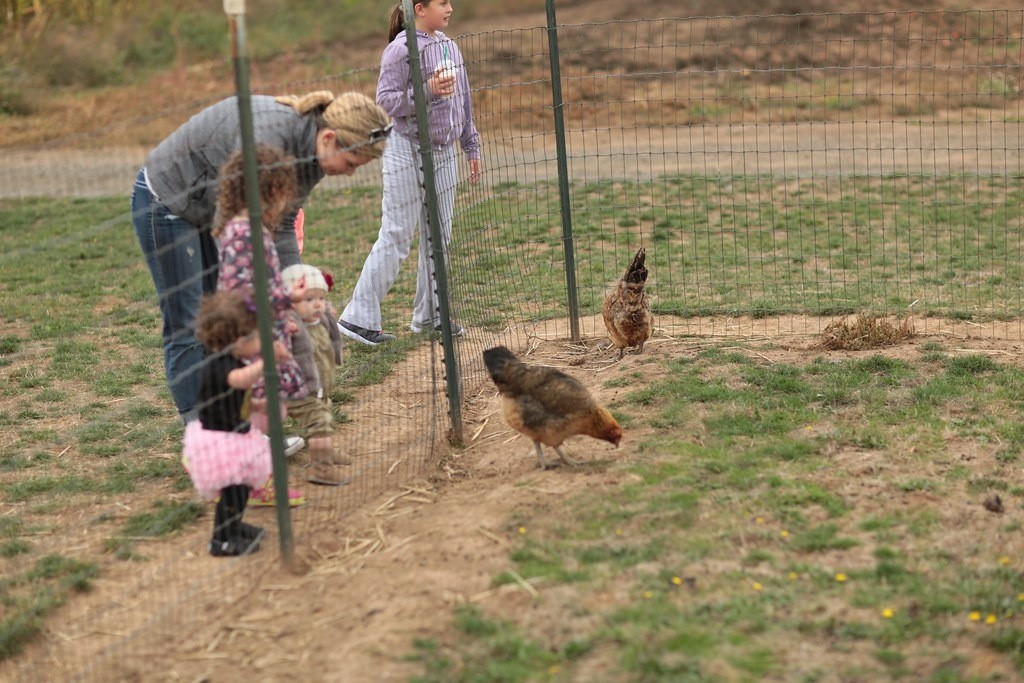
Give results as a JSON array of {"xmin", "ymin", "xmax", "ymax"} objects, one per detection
[{"xmin": 437, "ymin": 60, "xmax": 456, "ymax": 100}]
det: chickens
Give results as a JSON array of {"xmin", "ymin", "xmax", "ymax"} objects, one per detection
[
  {"xmin": 601, "ymin": 246, "xmax": 655, "ymax": 363},
  {"xmin": 482, "ymin": 345, "xmax": 623, "ymax": 474}
]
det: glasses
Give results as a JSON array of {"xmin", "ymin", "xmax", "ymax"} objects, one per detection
[{"xmin": 333, "ymin": 124, "xmax": 394, "ymax": 145}]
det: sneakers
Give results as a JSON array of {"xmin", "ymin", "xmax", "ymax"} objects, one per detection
[
  {"xmin": 410, "ymin": 322, "xmax": 463, "ymax": 336},
  {"xmin": 284, "ymin": 437, "xmax": 304, "ymax": 454},
  {"xmin": 337, "ymin": 320, "xmax": 396, "ymax": 346},
  {"xmin": 247, "ymin": 477, "xmax": 306, "ymax": 508}
]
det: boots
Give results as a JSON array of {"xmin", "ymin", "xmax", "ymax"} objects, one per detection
[
  {"xmin": 307, "ymin": 437, "xmax": 352, "ymax": 486},
  {"xmin": 210, "ymin": 505, "xmax": 266, "ymax": 558}
]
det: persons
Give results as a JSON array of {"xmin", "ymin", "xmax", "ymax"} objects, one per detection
[
  {"xmin": 337, "ymin": 0, "xmax": 483, "ymax": 345},
  {"xmin": 182, "ymin": 295, "xmax": 275, "ymax": 557},
  {"xmin": 130, "ymin": 90, "xmax": 391, "ymax": 430},
  {"xmin": 214, "ymin": 143, "xmax": 353, "ymax": 508}
]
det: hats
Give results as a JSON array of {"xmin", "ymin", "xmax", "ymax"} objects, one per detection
[{"xmin": 280, "ymin": 265, "xmax": 334, "ymax": 292}]
